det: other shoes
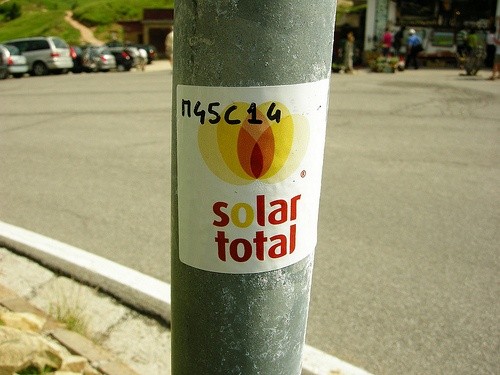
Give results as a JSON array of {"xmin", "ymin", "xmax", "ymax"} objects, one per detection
[
  {"xmin": 405, "ymin": 66, "xmax": 408, "ymax": 69},
  {"xmin": 414, "ymin": 66, "xmax": 418, "ymax": 68},
  {"xmin": 489, "ymin": 75, "xmax": 500, "ymax": 80}
]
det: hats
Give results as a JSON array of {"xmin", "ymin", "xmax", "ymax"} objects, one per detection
[{"xmin": 408, "ymin": 29, "xmax": 416, "ymax": 35}]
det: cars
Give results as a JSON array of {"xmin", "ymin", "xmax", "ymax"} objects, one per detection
[
  {"xmin": 0, "ymin": 44, "xmax": 28, "ymax": 80},
  {"xmin": 68, "ymin": 42, "xmax": 158, "ymax": 73}
]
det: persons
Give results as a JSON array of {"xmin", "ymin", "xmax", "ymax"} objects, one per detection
[{"xmin": 338, "ymin": 24, "xmax": 500, "ymax": 81}]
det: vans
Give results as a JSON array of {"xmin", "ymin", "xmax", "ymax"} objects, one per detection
[{"xmin": 3, "ymin": 36, "xmax": 73, "ymax": 76}]
